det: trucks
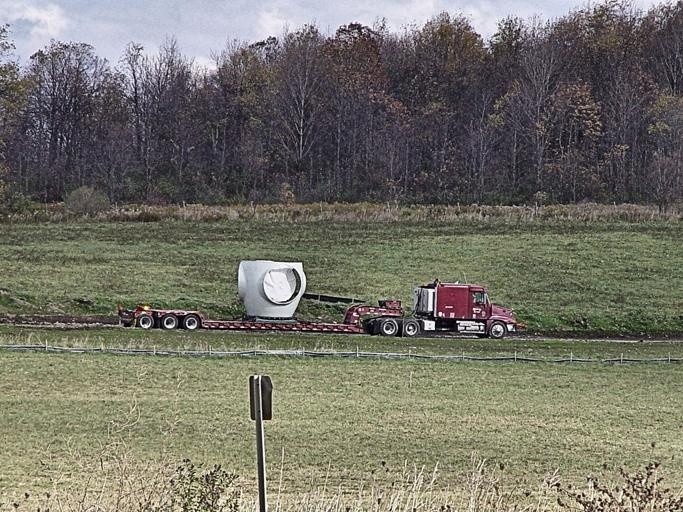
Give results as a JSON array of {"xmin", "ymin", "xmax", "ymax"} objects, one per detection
[{"xmin": 362, "ymin": 278, "xmax": 517, "ymax": 339}]
